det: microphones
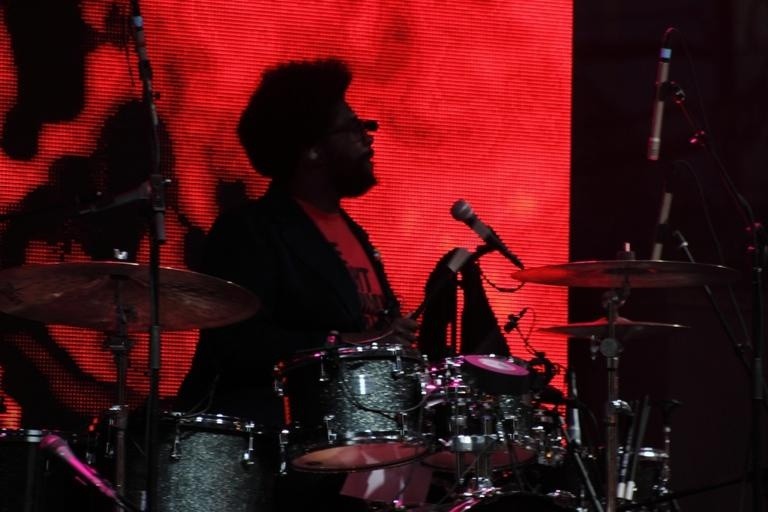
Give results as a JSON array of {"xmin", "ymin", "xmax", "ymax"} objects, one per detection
[
  {"xmin": 649, "ymin": 28, "xmax": 675, "ymax": 161},
  {"xmin": 505, "ymin": 308, "xmax": 526, "ymax": 332},
  {"xmin": 652, "ymin": 163, "xmax": 679, "ymax": 261},
  {"xmin": 74, "ymin": 181, "xmax": 156, "ymax": 218},
  {"xmin": 451, "ymin": 201, "xmax": 522, "ymax": 274}
]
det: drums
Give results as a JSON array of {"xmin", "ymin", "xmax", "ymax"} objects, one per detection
[
  {"xmin": 3, "ymin": 427, "xmax": 105, "ymax": 511},
  {"xmin": 452, "ymin": 485, "xmax": 588, "ymax": 512},
  {"xmin": 106, "ymin": 407, "xmax": 272, "ymax": 511},
  {"xmin": 272, "ymin": 343, "xmax": 436, "ymax": 475},
  {"xmin": 421, "ymin": 355, "xmax": 542, "ymax": 468}
]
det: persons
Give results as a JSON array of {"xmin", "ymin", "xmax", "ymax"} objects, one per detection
[{"xmin": 177, "ymin": 52, "xmax": 421, "ymax": 445}]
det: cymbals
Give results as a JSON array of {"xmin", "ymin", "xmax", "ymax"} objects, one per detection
[
  {"xmin": 536, "ymin": 317, "xmax": 688, "ymax": 337},
  {"xmin": 0, "ymin": 262, "xmax": 259, "ymax": 333},
  {"xmin": 512, "ymin": 259, "xmax": 740, "ymax": 288}
]
